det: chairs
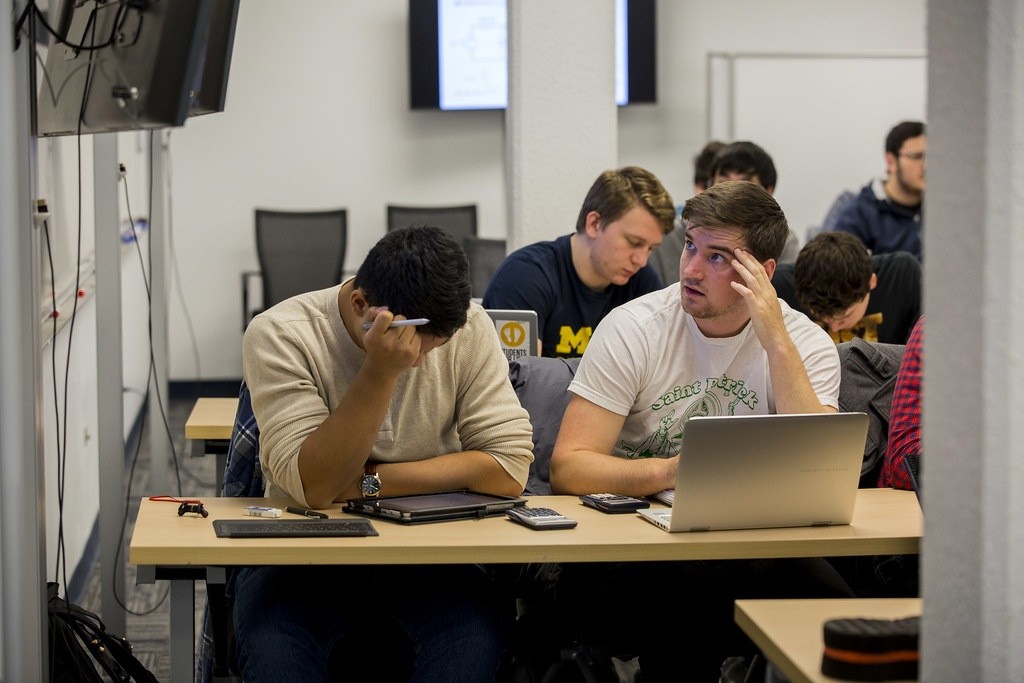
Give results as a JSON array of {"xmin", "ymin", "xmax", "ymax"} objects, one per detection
[{"xmin": 241, "ymin": 203, "xmax": 506, "ymax": 336}]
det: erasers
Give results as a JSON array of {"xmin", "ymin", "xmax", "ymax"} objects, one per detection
[{"xmin": 243, "ymin": 505, "xmax": 282, "ymax": 518}]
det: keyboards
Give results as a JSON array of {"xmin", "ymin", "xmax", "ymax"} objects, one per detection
[{"xmin": 213, "ymin": 519, "xmax": 379, "ymax": 538}]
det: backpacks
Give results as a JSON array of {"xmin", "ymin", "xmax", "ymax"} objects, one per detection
[{"xmin": 48, "ymin": 583, "xmax": 159, "ymax": 683}]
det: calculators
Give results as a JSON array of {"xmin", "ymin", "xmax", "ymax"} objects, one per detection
[
  {"xmin": 579, "ymin": 493, "xmax": 650, "ymax": 513},
  {"xmin": 506, "ymin": 508, "xmax": 578, "ymax": 530}
]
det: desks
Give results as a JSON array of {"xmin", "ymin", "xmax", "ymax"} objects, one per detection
[
  {"xmin": 184, "ymin": 397, "xmax": 241, "ymax": 497},
  {"xmin": 129, "ymin": 487, "xmax": 924, "ymax": 683},
  {"xmin": 733, "ymin": 597, "xmax": 923, "ymax": 683}
]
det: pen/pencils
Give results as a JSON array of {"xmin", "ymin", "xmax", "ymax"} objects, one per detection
[
  {"xmin": 363, "ymin": 317, "xmax": 430, "ymax": 330},
  {"xmin": 285, "ymin": 506, "xmax": 329, "ymax": 519}
]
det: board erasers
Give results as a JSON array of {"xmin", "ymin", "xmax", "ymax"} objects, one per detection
[{"xmin": 822, "ymin": 616, "xmax": 919, "ymax": 679}]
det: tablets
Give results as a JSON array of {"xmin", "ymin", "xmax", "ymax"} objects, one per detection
[{"xmin": 342, "ymin": 488, "xmax": 528, "ymax": 523}]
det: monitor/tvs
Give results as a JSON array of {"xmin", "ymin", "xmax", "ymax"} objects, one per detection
[{"xmin": 37, "ymin": 0, "xmax": 240, "ymax": 136}]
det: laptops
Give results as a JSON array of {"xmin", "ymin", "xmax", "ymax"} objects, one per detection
[
  {"xmin": 486, "ymin": 309, "xmax": 538, "ymax": 362},
  {"xmin": 636, "ymin": 412, "xmax": 871, "ymax": 533}
]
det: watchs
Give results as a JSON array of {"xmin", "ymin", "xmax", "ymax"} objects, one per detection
[{"xmin": 357, "ymin": 462, "xmax": 382, "ymax": 499}]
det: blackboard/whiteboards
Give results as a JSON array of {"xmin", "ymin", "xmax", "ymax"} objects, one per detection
[{"xmin": 704, "ymin": 52, "xmax": 926, "ymax": 250}]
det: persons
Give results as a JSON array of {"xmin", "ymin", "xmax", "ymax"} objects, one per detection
[
  {"xmin": 232, "ymin": 225, "xmax": 535, "ymax": 683},
  {"xmin": 549, "ymin": 180, "xmax": 842, "ymax": 498},
  {"xmin": 482, "ymin": 167, "xmax": 676, "ymax": 359},
  {"xmin": 771, "ymin": 232, "xmax": 922, "ymax": 345},
  {"xmin": 834, "ymin": 122, "xmax": 927, "ymax": 264},
  {"xmin": 648, "ymin": 142, "xmax": 799, "ymax": 289}
]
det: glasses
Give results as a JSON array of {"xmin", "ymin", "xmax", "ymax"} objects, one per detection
[{"xmin": 898, "ymin": 152, "xmax": 924, "ymax": 160}]
